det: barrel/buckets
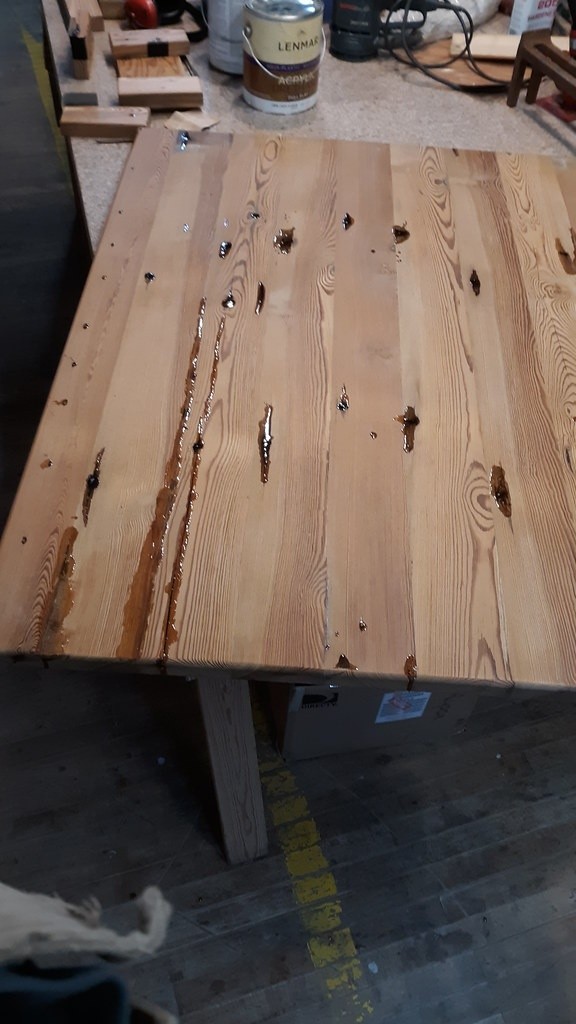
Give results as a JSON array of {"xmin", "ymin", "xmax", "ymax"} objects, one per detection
[{"xmin": 200, "ymin": 1, "xmax": 376, "ymax": 114}]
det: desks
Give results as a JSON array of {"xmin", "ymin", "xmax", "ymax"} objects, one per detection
[{"xmin": 40, "ymin": 1, "xmax": 575, "ymax": 863}]
